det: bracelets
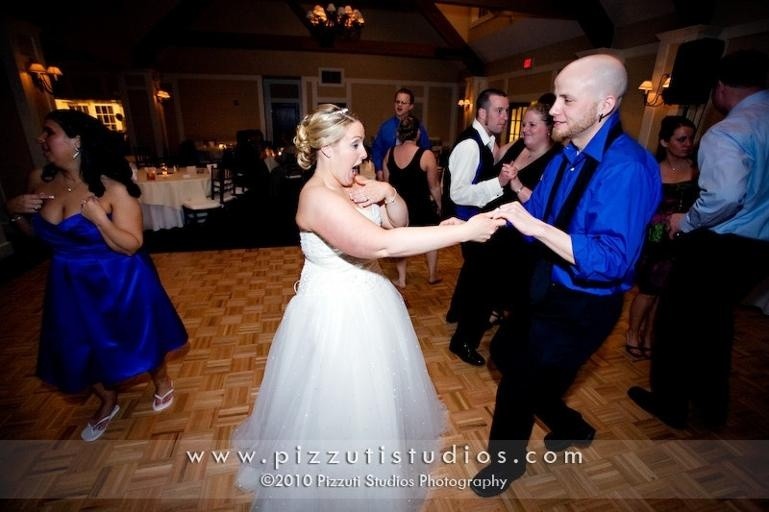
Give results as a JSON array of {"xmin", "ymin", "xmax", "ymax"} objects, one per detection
[{"xmin": 11, "ymin": 215, "xmax": 24, "ymax": 222}]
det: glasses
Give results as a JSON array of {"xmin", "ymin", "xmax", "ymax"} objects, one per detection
[{"xmin": 396, "ymin": 101, "xmax": 410, "ymax": 104}]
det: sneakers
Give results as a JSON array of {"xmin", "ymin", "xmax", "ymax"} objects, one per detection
[{"xmin": 629, "ymin": 386, "xmax": 687, "ymax": 429}]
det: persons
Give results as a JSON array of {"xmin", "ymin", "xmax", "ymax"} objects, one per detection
[
  {"xmin": 6, "ymin": 109, "xmax": 188, "ymax": 431},
  {"xmin": 230, "ymin": 48, "xmax": 769, "ymax": 512}
]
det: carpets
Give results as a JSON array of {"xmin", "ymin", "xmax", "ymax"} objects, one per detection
[{"xmin": 144, "ymin": 172, "xmax": 440, "ymax": 254}]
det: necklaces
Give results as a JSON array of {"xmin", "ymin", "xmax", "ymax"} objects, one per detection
[{"xmin": 64, "ymin": 184, "xmax": 72, "ymax": 192}]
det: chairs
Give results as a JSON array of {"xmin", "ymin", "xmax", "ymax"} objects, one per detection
[{"xmin": 124, "ymin": 139, "xmax": 444, "ymax": 232}]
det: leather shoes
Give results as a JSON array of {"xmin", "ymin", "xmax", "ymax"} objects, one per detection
[
  {"xmin": 544, "ymin": 420, "xmax": 596, "ymax": 451},
  {"xmin": 469, "ymin": 462, "xmax": 526, "ymax": 496},
  {"xmin": 449, "ymin": 343, "xmax": 485, "ymax": 366}
]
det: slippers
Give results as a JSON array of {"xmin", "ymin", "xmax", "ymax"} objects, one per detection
[
  {"xmin": 625, "ymin": 345, "xmax": 651, "ymax": 358},
  {"xmin": 80, "ymin": 405, "xmax": 120, "ymax": 441},
  {"xmin": 153, "ymin": 380, "xmax": 174, "ymax": 410}
]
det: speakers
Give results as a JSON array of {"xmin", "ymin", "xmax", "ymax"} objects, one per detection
[{"xmin": 661, "ymin": 37, "xmax": 724, "ymax": 107}]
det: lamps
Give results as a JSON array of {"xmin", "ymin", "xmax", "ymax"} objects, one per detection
[
  {"xmin": 28, "ymin": 61, "xmax": 63, "ymax": 93},
  {"xmin": 637, "ymin": 72, "xmax": 672, "ymax": 107},
  {"xmin": 455, "ymin": 95, "xmax": 471, "ymax": 109},
  {"xmin": 306, "ymin": 0, "xmax": 364, "ymax": 29},
  {"xmin": 157, "ymin": 89, "xmax": 171, "ymax": 102}
]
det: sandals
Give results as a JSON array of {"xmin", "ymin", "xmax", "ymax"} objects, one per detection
[{"xmin": 486, "ymin": 311, "xmax": 503, "ymax": 328}]
